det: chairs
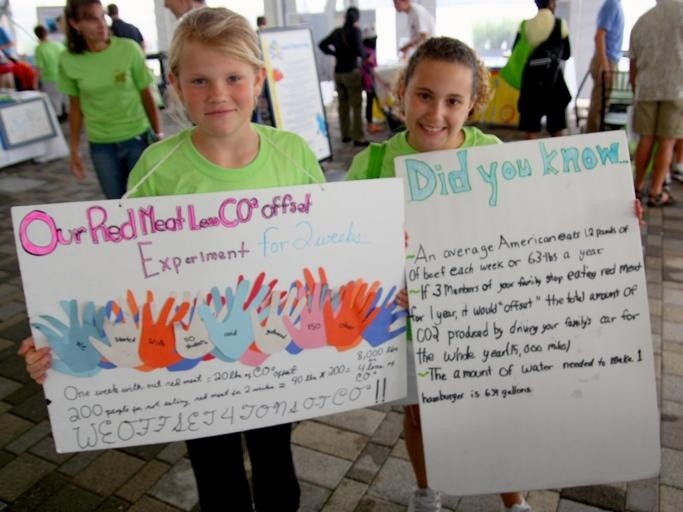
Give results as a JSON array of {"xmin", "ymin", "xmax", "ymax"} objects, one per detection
[{"xmin": 600, "ymin": 70, "xmax": 634, "ymax": 131}]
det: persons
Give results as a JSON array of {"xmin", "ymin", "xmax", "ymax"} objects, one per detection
[
  {"xmin": 512, "ymin": 1, "xmax": 572, "ymax": 140},
  {"xmin": 33, "ymin": 1, "xmax": 165, "ymax": 201},
  {"xmin": 319, "ymin": 7, "xmax": 383, "ymax": 146},
  {"xmin": 250, "ymin": 17, "xmax": 273, "ymax": 128},
  {"xmin": 0, "ymin": 26, "xmax": 14, "ymax": 66},
  {"xmin": 587, "ymin": 1, "xmax": 625, "ymax": 133},
  {"xmin": 341, "ymin": 36, "xmax": 645, "ymax": 511},
  {"xmin": 16, "ymin": 6, "xmax": 409, "ymax": 512},
  {"xmin": 629, "ymin": 1, "xmax": 682, "ymax": 206},
  {"xmin": 394, "ymin": 0, "xmax": 435, "ymax": 53}
]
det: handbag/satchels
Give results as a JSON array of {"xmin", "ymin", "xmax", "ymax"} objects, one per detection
[
  {"xmin": 499, "ymin": 20, "xmax": 535, "ymax": 90},
  {"xmin": 525, "ymin": 19, "xmax": 565, "ymax": 88}
]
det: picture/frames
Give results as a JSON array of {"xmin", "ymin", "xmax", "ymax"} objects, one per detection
[{"xmin": 256, "ymin": 27, "xmax": 333, "ymax": 167}]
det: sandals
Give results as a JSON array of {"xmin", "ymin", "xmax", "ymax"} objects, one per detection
[{"xmin": 646, "ymin": 191, "xmax": 677, "ymax": 207}]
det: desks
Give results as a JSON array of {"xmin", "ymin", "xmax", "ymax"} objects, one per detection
[
  {"xmin": 371, "ymin": 65, "xmax": 523, "ymax": 132},
  {"xmin": 0, "ymin": 86, "xmax": 70, "ymax": 172}
]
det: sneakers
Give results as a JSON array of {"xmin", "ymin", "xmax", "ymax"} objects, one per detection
[
  {"xmin": 407, "ymin": 484, "xmax": 442, "ymax": 511},
  {"xmin": 342, "ymin": 123, "xmax": 384, "ymax": 147}
]
